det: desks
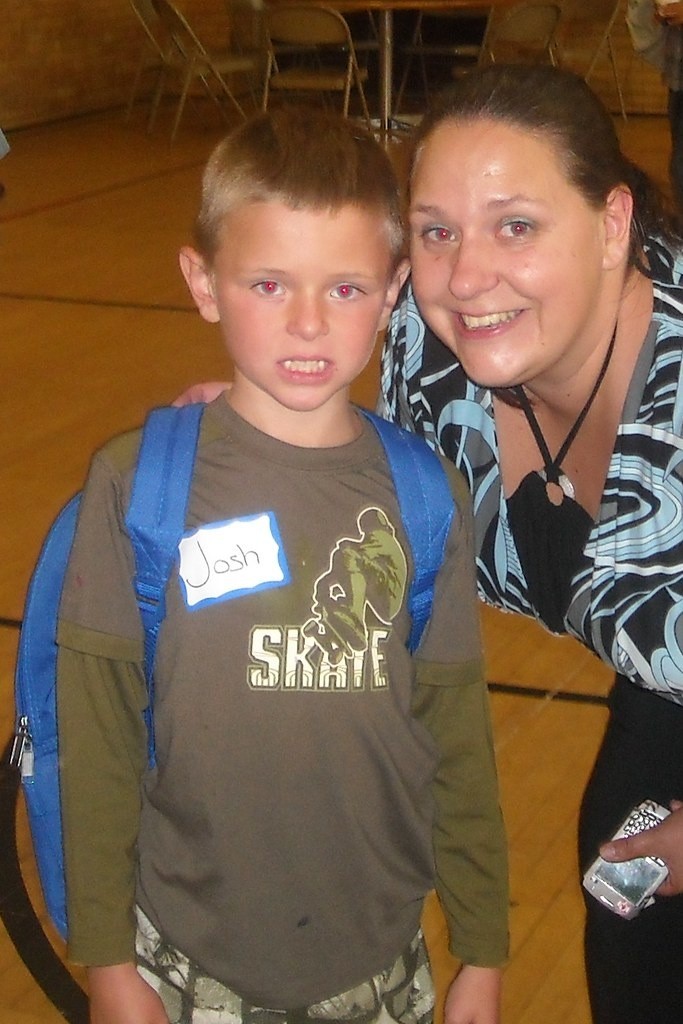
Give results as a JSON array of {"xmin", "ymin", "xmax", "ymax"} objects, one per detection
[{"xmin": 231, "ymin": 1, "xmax": 495, "ymax": 156}]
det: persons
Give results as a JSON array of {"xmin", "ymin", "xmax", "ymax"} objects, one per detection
[
  {"xmin": 651, "ymin": 0, "xmax": 683, "ymax": 213},
  {"xmin": 8, "ymin": 100, "xmax": 513, "ymax": 1021},
  {"xmin": 168, "ymin": 58, "xmax": 683, "ymax": 1024}
]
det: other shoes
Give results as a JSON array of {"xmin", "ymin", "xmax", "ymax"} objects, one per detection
[{"xmin": 666, "ymin": 214, "xmax": 683, "ymax": 244}]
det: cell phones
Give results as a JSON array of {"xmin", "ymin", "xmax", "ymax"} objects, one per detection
[{"xmin": 582, "ymin": 800, "xmax": 673, "ymax": 920}]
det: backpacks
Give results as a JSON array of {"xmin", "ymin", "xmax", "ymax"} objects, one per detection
[{"xmin": 10, "ymin": 401, "xmax": 459, "ymax": 939}]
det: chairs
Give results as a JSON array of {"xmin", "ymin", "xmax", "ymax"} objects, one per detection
[
  {"xmin": 551, "ymin": 0, "xmax": 627, "ymax": 122},
  {"xmin": 144, "ymin": 0, "xmax": 262, "ymax": 144},
  {"xmin": 118, "ymin": 0, "xmax": 230, "ymax": 130},
  {"xmin": 476, "ymin": 1, "xmax": 562, "ymax": 75},
  {"xmin": 392, "ymin": 0, "xmax": 491, "ymax": 122},
  {"xmin": 259, "ymin": 3, "xmax": 374, "ymax": 134}
]
